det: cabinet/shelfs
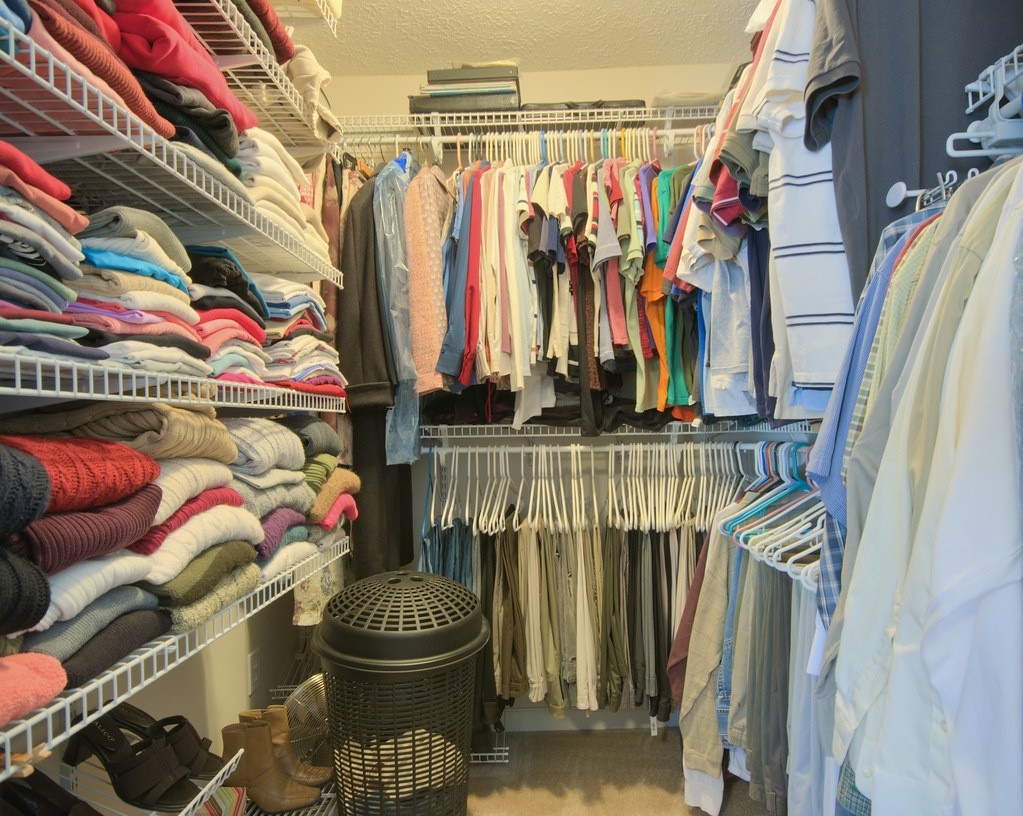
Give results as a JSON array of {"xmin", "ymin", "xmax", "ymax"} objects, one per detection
[{"xmin": 0, "ymin": 0, "xmax": 349, "ymax": 816}]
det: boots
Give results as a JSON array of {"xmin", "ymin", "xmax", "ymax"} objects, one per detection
[
  {"xmin": 220, "ymin": 721, "xmax": 321, "ymax": 814},
  {"xmin": 238, "ymin": 705, "xmax": 334, "ymax": 787}
]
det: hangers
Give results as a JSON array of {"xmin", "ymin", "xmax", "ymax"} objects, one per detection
[
  {"xmin": 419, "ymin": 442, "xmax": 826, "ymax": 591},
  {"xmin": 328, "ymin": 125, "xmax": 719, "ymax": 178}
]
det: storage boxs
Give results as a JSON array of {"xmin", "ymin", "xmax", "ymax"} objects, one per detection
[{"xmin": 520, "ymin": 98, "xmax": 647, "ymax": 130}]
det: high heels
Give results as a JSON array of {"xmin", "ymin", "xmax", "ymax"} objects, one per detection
[{"xmin": 97, "ymin": 698, "xmax": 226, "ymax": 780}]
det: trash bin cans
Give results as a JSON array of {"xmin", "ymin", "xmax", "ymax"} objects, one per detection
[{"xmin": 308, "ymin": 570, "xmax": 491, "ymax": 816}]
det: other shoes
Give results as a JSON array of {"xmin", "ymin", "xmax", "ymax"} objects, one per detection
[{"xmin": 62, "ymin": 709, "xmax": 204, "ymax": 813}]
